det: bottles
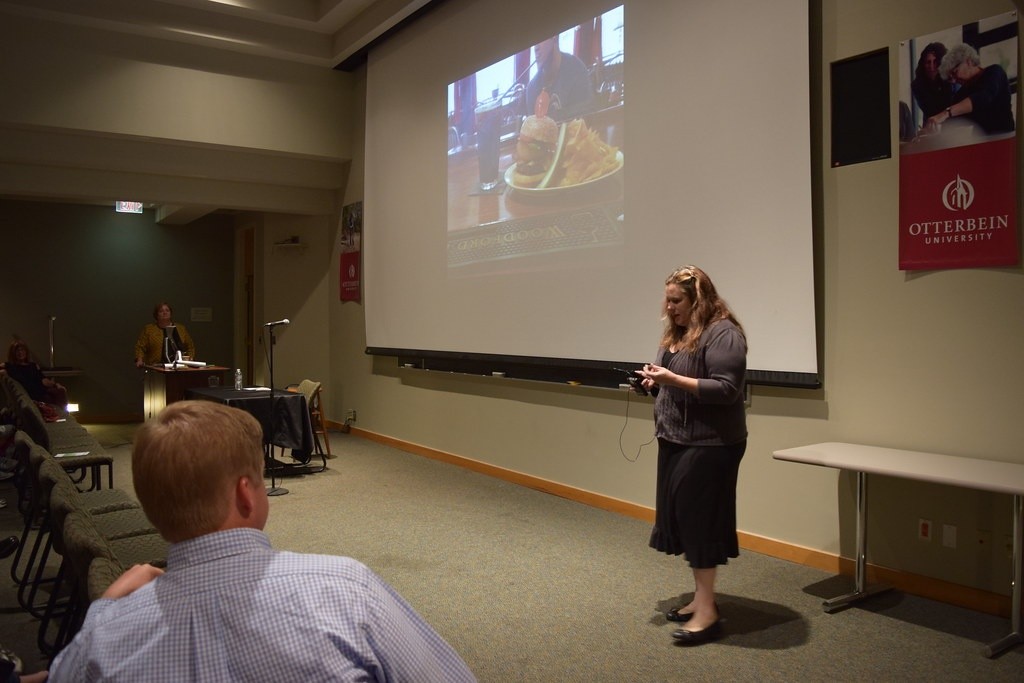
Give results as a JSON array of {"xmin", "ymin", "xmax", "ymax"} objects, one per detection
[{"xmin": 235, "ymin": 368, "xmax": 242, "ymax": 390}]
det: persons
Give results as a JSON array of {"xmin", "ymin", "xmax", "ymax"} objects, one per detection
[
  {"xmin": 47, "ymin": 400, "xmax": 478, "ymax": 683},
  {"xmin": 912, "ymin": 41, "xmax": 961, "ymax": 131},
  {"xmin": 924, "ymin": 42, "xmax": 1015, "ymax": 136},
  {"xmin": 135, "ymin": 306, "xmax": 193, "ymax": 367},
  {"xmin": 525, "ymin": 33, "xmax": 595, "ymax": 123},
  {"xmin": 0, "ymin": 648, "xmax": 49, "ymax": 683},
  {"xmin": 627, "ymin": 264, "xmax": 751, "ymax": 646},
  {"xmin": 0, "ymin": 536, "xmax": 19, "ymax": 560},
  {"xmin": 6, "ymin": 339, "xmax": 67, "ymax": 410}
]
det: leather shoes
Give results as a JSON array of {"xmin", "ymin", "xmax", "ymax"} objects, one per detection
[
  {"xmin": 670, "ymin": 618, "xmax": 721, "ymax": 641},
  {"xmin": 666, "ymin": 601, "xmax": 719, "ymax": 622}
]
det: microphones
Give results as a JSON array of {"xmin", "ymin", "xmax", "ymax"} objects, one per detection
[{"xmin": 264, "ymin": 318, "xmax": 290, "ymax": 327}]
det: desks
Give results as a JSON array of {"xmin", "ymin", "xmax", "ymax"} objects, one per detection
[
  {"xmin": 772, "ymin": 441, "xmax": 1024, "ymax": 659},
  {"xmin": 186, "ymin": 385, "xmax": 305, "ymax": 479}
]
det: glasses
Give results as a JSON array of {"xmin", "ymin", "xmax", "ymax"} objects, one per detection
[{"xmin": 947, "ymin": 61, "xmax": 965, "ymax": 80}]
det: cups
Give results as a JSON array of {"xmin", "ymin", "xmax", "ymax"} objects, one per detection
[{"xmin": 474, "ymin": 97, "xmax": 504, "ymax": 190}]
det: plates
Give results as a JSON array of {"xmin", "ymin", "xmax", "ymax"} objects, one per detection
[{"xmin": 502, "ymin": 151, "xmax": 625, "ymax": 197}]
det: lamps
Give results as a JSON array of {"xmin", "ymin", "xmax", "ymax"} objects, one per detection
[{"xmin": 115, "ymin": 201, "xmax": 144, "ymax": 214}]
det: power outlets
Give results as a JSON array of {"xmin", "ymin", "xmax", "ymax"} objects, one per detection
[{"xmin": 346, "ymin": 409, "xmax": 353, "ymax": 419}]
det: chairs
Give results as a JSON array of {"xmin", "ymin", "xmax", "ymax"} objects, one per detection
[
  {"xmin": 281, "ymin": 379, "xmax": 333, "ymax": 465},
  {"xmin": 502, "ymin": 83, "xmax": 524, "ymax": 133},
  {"xmin": 9, "ymin": 432, "xmax": 176, "ymax": 670},
  {"xmin": 3, "ymin": 374, "xmax": 115, "ymax": 532},
  {"xmin": 447, "ymin": 126, "xmax": 459, "ymax": 147}
]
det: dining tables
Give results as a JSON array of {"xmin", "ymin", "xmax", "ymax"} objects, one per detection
[{"xmin": 447, "ymin": 105, "xmax": 624, "ymax": 232}]
